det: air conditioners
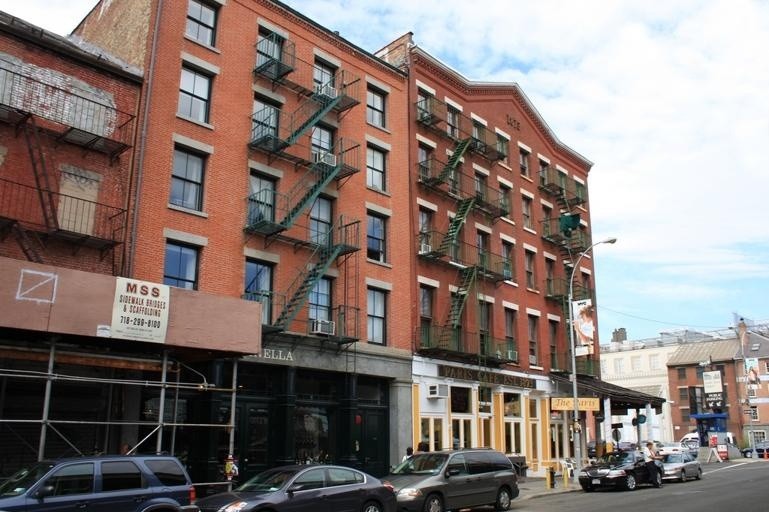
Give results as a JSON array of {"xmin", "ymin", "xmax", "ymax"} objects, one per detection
[
  {"xmin": 314, "ymin": 151, "xmax": 336, "ymax": 167},
  {"xmin": 427, "ymin": 384, "xmax": 449, "ymax": 398},
  {"xmin": 316, "ymin": 85, "xmax": 338, "ymax": 99},
  {"xmin": 311, "ymin": 320, "xmax": 335, "ymax": 335},
  {"xmin": 418, "ymin": 243, "xmax": 431, "ymax": 254},
  {"xmin": 504, "ymin": 350, "xmax": 517, "ymax": 360}
]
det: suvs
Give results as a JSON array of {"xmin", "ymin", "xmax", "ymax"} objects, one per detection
[{"xmin": 0, "ymin": 453, "xmax": 200, "ymax": 512}]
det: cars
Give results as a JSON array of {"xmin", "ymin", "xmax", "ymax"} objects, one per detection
[
  {"xmin": 655, "ymin": 450, "xmax": 703, "ymax": 483},
  {"xmin": 195, "ymin": 465, "xmax": 400, "ymax": 512},
  {"xmin": 579, "ymin": 449, "xmax": 667, "ymax": 493},
  {"xmin": 741, "ymin": 441, "xmax": 769, "ymax": 459},
  {"xmin": 587, "ymin": 437, "xmax": 703, "ymax": 461}
]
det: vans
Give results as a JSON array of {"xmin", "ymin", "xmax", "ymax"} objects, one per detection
[
  {"xmin": 679, "ymin": 432, "xmax": 740, "ymax": 452},
  {"xmin": 378, "ymin": 449, "xmax": 521, "ymax": 512}
]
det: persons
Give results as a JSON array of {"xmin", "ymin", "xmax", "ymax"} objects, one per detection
[
  {"xmin": 401, "ymin": 445, "xmax": 414, "ymax": 470},
  {"xmin": 643, "ymin": 441, "xmax": 665, "ymax": 489},
  {"xmin": 119, "ymin": 441, "xmax": 136, "ymax": 456},
  {"xmin": 573, "ymin": 304, "xmax": 594, "ymax": 347},
  {"xmin": 747, "ymin": 365, "xmax": 758, "ymax": 384}
]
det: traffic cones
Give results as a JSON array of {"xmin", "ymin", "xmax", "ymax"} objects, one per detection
[{"xmin": 764, "ymin": 448, "xmax": 769, "ymax": 460}]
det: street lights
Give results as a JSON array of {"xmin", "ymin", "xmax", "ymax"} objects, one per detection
[
  {"xmin": 741, "ymin": 329, "xmax": 759, "ymax": 459},
  {"xmin": 567, "ymin": 237, "xmax": 617, "ymax": 492}
]
